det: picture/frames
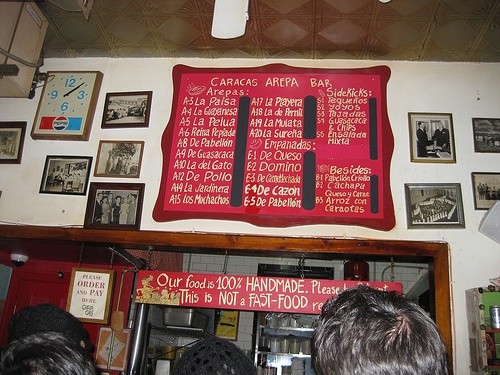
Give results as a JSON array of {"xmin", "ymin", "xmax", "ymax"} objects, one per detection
[
  {"xmin": 472, "ymin": 172, "xmax": 500, "ymax": 210},
  {"xmin": 472, "ymin": 118, "xmax": 500, "ymax": 153},
  {"xmin": 404, "ymin": 183, "xmax": 466, "ymax": 229},
  {"xmin": 0, "ymin": 121, "xmax": 27, "ymax": 164},
  {"xmin": 39, "ymin": 155, "xmax": 93, "ymax": 195},
  {"xmin": 93, "ymin": 140, "xmax": 145, "ymax": 178},
  {"xmin": 407, "ymin": 112, "xmax": 456, "ymax": 163},
  {"xmin": 83, "ymin": 182, "xmax": 145, "ymax": 230},
  {"xmin": 101, "ymin": 91, "xmax": 153, "ymax": 128}
]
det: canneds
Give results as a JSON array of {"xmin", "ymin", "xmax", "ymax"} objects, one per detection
[{"xmin": 491, "ymin": 305, "xmax": 500, "ymax": 329}]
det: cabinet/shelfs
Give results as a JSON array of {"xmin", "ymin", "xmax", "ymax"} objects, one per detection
[{"xmin": 254, "ymin": 312, "xmax": 315, "ymax": 375}]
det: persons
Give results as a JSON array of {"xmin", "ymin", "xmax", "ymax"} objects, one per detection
[
  {"xmin": 0, "ymin": 302, "xmax": 97, "ymax": 375},
  {"xmin": 478, "ymin": 182, "xmax": 500, "ymax": 200},
  {"xmin": 47, "ymin": 169, "xmax": 83, "ymax": 190},
  {"xmin": 93, "ymin": 192, "xmax": 136, "ymax": 224},
  {"xmin": 310, "ymin": 283, "xmax": 449, "ymax": 375},
  {"xmin": 417, "ymin": 122, "xmax": 450, "ymax": 157}
]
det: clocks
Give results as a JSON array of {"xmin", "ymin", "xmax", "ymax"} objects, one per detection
[{"xmin": 30, "ymin": 71, "xmax": 103, "ymax": 141}]
